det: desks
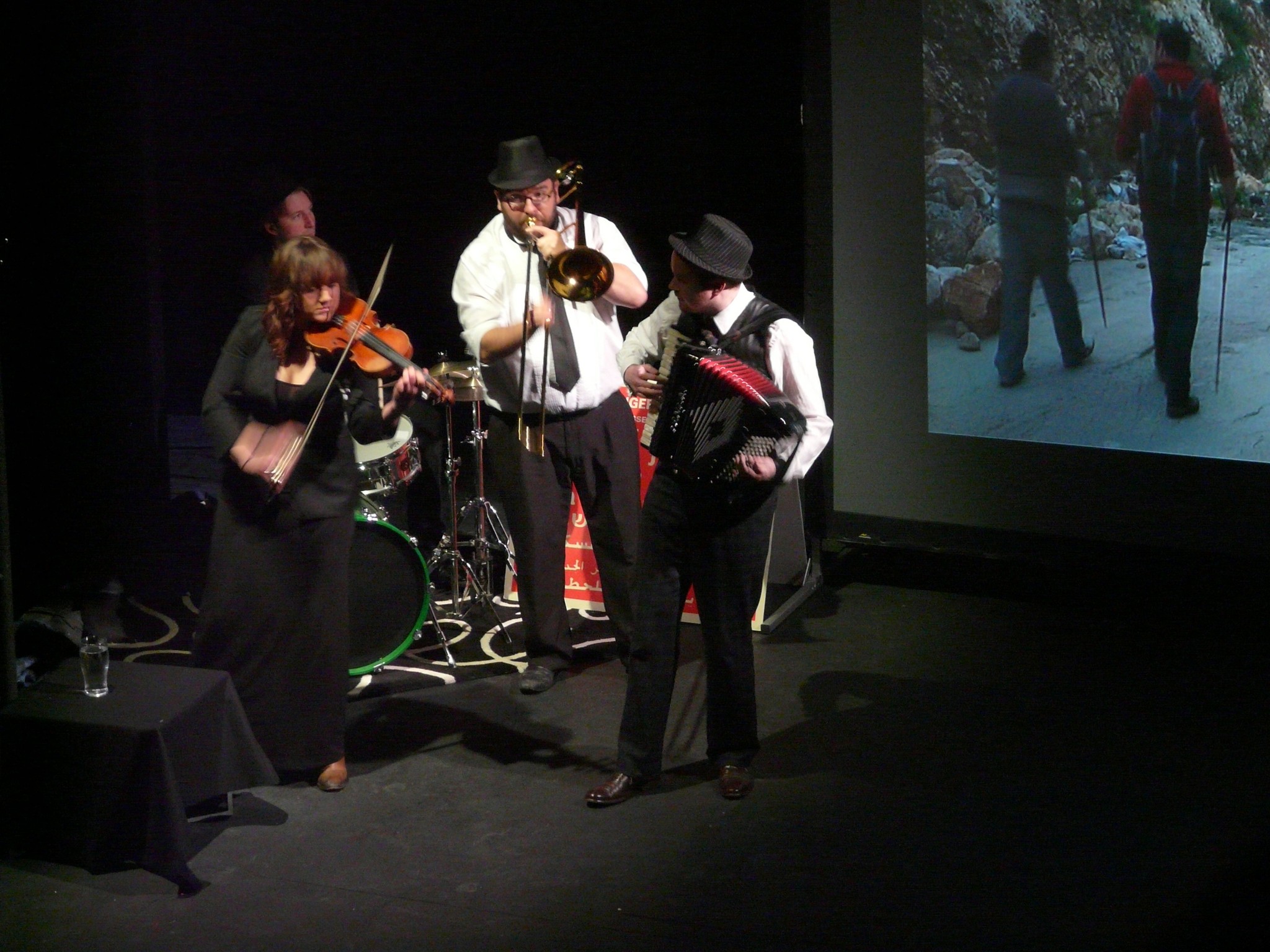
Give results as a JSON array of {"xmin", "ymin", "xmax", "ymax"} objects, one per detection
[{"xmin": 1, "ymin": 654, "xmax": 283, "ymax": 902}]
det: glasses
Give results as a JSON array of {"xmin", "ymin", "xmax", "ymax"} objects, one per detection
[{"xmin": 496, "ymin": 184, "xmax": 554, "ymax": 211}]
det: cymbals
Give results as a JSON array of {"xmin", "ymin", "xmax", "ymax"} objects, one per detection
[
  {"xmin": 453, "ymin": 372, "xmax": 488, "ymax": 402},
  {"xmin": 427, "ymin": 351, "xmax": 477, "ymax": 377}
]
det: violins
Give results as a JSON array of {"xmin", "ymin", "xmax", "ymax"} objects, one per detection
[{"xmin": 303, "ymin": 291, "xmax": 453, "ymax": 405}]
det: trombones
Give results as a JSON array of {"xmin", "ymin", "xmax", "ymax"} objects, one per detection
[{"xmin": 518, "ymin": 162, "xmax": 615, "ymax": 456}]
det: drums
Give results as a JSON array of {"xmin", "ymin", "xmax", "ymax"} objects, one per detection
[
  {"xmin": 351, "ymin": 413, "xmax": 421, "ymax": 497},
  {"xmin": 349, "ymin": 513, "xmax": 434, "ymax": 676}
]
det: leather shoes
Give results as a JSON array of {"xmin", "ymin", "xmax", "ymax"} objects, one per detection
[
  {"xmin": 582, "ymin": 773, "xmax": 643, "ymax": 805},
  {"xmin": 518, "ymin": 664, "xmax": 554, "ymax": 695},
  {"xmin": 316, "ymin": 755, "xmax": 348, "ymax": 789},
  {"xmin": 717, "ymin": 759, "xmax": 754, "ymax": 799}
]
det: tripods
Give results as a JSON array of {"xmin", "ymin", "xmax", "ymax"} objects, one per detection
[{"xmin": 416, "ymin": 396, "xmax": 524, "ymax": 667}]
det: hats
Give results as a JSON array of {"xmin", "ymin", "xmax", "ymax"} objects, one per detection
[
  {"xmin": 669, "ymin": 213, "xmax": 754, "ymax": 280},
  {"xmin": 488, "ymin": 135, "xmax": 561, "ymax": 190}
]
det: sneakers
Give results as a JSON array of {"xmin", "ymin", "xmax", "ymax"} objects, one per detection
[
  {"xmin": 999, "ymin": 367, "xmax": 1024, "ymax": 384},
  {"xmin": 1060, "ymin": 333, "xmax": 1095, "ymax": 365},
  {"xmin": 1166, "ymin": 396, "xmax": 1199, "ymax": 418}
]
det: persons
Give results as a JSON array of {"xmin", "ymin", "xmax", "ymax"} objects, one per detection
[
  {"xmin": 1114, "ymin": 19, "xmax": 1244, "ymax": 418},
  {"xmin": 253, "ymin": 185, "xmax": 316, "ymax": 244},
  {"xmin": 185, "ymin": 237, "xmax": 432, "ymax": 793},
  {"xmin": 986, "ymin": 30, "xmax": 1099, "ymax": 386},
  {"xmin": 452, "ymin": 133, "xmax": 652, "ymax": 690},
  {"xmin": 583, "ymin": 212, "xmax": 833, "ymax": 805}
]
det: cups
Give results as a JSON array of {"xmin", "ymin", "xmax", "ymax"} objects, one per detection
[{"xmin": 80, "ymin": 635, "xmax": 110, "ymax": 697}]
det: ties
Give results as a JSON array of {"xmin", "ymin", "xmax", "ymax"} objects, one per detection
[{"xmin": 538, "ymin": 251, "xmax": 580, "ymax": 394}]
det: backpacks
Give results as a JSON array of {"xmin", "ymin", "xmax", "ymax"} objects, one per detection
[{"xmin": 1136, "ymin": 66, "xmax": 1213, "ymax": 213}]
